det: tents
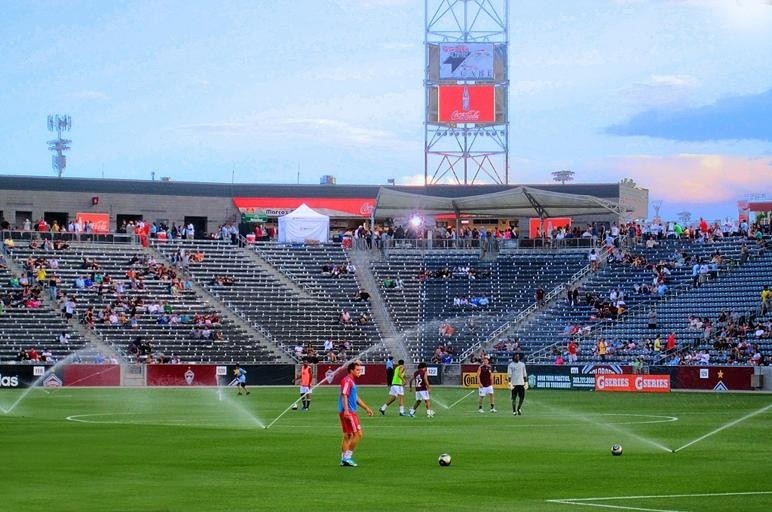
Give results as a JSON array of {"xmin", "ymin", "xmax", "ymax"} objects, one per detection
[{"xmin": 277, "ymin": 203, "xmax": 330, "ymax": 242}]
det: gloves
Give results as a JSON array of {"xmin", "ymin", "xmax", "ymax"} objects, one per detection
[
  {"xmin": 524, "ymin": 382, "xmax": 528, "ymax": 389},
  {"xmin": 508, "ymin": 383, "xmax": 514, "ymax": 390}
]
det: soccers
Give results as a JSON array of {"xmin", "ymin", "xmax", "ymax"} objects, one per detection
[
  {"xmin": 439, "ymin": 454, "xmax": 451, "ymax": 466},
  {"xmin": 611, "ymin": 444, "xmax": 622, "ymax": 455},
  {"xmin": 292, "ymin": 404, "xmax": 298, "ymax": 410}
]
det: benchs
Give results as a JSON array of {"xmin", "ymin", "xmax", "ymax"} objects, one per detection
[{"xmin": 0, "ymin": 234, "xmax": 772, "ymax": 366}]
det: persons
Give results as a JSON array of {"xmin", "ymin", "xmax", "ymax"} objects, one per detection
[
  {"xmin": 336, "ymin": 361, "xmax": 373, "ymax": 467},
  {"xmin": 384, "ymin": 353, "xmax": 394, "ymax": 386},
  {"xmin": 292, "ymin": 359, "xmax": 314, "ymax": 410},
  {"xmin": 409, "ymin": 362, "xmax": 436, "ymax": 418},
  {"xmin": 476, "ymin": 356, "xmax": 498, "ymax": 413},
  {"xmin": 378, "ymin": 359, "xmax": 408, "ymax": 417},
  {"xmin": 1, "ymin": 217, "xmax": 772, "ymax": 366},
  {"xmin": 233, "ymin": 363, "xmax": 251, "ymax": 396},
  {"xmin": 506, "ymin": 352, "xmax": 528, "ymax": 416}
]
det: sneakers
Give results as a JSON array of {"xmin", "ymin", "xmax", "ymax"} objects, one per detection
[
  {"xmin": 399, "ymin": 412, "xmax": 406, "ymax": 416},
  {"xmin": 427, "ymin": 414, "xmax": 433, "ymax": 418},
  {"xmin": 342, "ymin": 457, "xmax": 357, "ymax": 466},
  {"xmin": 478, "ymin": 409, "xmax": 522, "ymax": 416},
  {"xmin": 379, "ymin": 408, "xmax": 385, "ymax": 415},
  {"xmin": 340, "ymin": 459, "xmax": 345, "ymax": 466},
  {"xmin": 409, "ymin": 413, "xmax": 416, "ymax": 418}
]
